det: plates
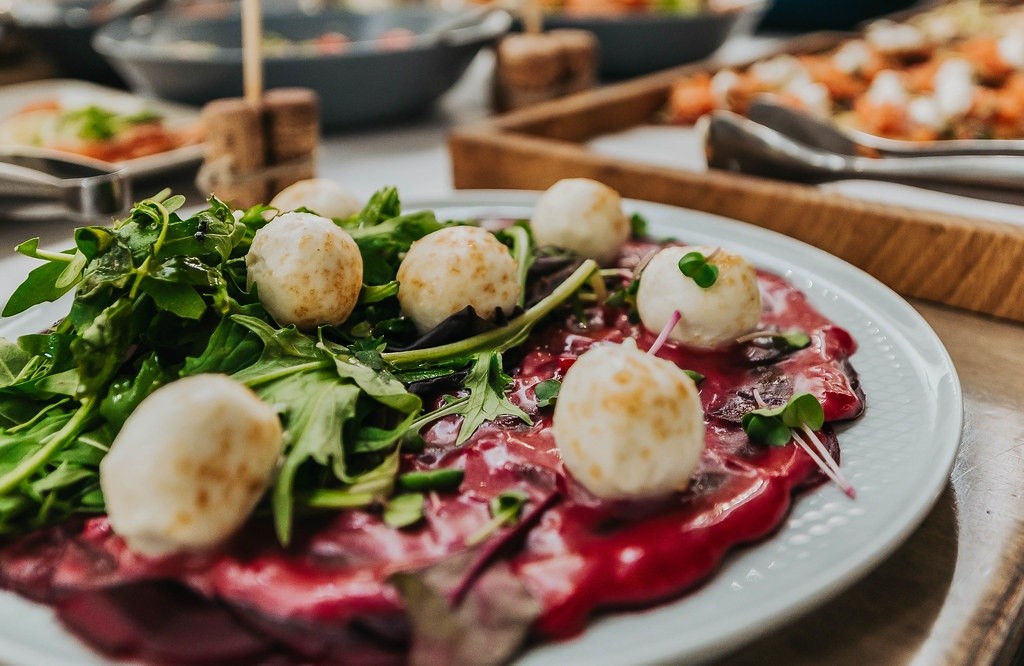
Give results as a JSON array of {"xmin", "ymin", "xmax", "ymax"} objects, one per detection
[
  {"xmin": 0, "ymin": 78, "xmax": 206, "ymax": 173},
  {"xmin": 0, "ymin": 189, "xmax": 965, "ymax": 666}
]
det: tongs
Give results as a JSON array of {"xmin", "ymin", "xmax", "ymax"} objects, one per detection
[
  {"xmin": 705, "ymin": 94, "xmax": 1024, "ymax": 189},
  {"xmin": 0, "ymin": 148, "xmax": 120, "ymax": 226}
]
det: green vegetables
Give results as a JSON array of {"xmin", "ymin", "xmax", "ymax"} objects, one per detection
[{"xmin": 1, "ymin": 188, "xmax": 596, "ymax": 554}]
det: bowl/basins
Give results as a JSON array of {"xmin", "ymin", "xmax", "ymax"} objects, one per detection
[
  {"xmin": 503, "ymin": 6, "xmax": 741, "ymax": 82},
  {"xmin": 91, "ymin": 0, "xmax": 512, "ymax": 128}
]
